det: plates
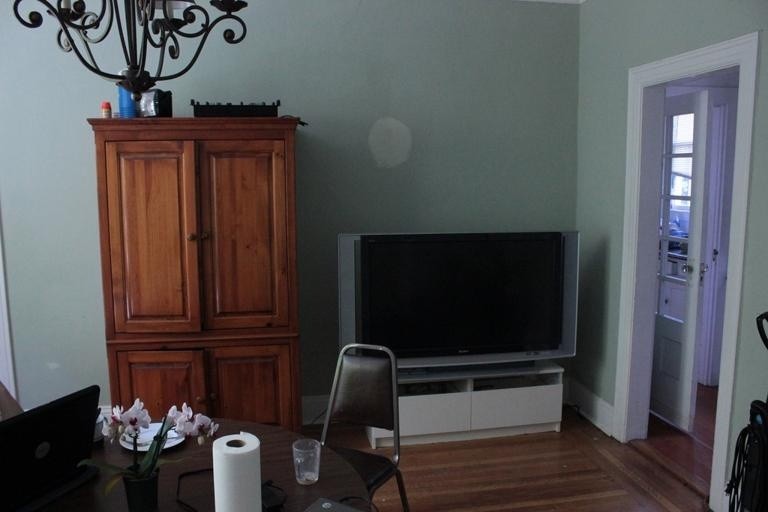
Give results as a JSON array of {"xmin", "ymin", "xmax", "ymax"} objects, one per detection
[{"xmin": 118, "ymin": 422, "xmax": 185, "ymax": 453}]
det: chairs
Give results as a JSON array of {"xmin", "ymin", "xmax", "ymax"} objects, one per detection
[{"xmin": 318, "ymin": 341, "xmax": 409, "ymax": 512}]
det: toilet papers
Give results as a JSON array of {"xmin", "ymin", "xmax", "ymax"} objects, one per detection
[{"xmin": 213, "ymin": 431, "xmax": 261, "ymax": 512}]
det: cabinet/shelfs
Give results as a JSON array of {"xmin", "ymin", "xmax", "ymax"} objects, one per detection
[
  {"xmin": 365, "ymin": 357, "xmax": 567, "ymax": 449},
  {"xmin": 86, "ymin": 115, "xmax": 304, "ymax": 435}
]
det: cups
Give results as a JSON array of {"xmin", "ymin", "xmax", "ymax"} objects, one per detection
[{"xmin": 291, "ymin": 436, "xmax": 321, "ymax": 486}]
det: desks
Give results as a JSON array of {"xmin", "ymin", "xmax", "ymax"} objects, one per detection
[{"xmin": 30, "ymin": 417, "xmax": 371, "ymax": 512}]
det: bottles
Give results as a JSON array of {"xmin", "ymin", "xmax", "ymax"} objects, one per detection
[{"xmin": 117, "ymin": 67, "xmax": 138, "ymax": 119}]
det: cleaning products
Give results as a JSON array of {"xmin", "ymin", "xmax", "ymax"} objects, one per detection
[{"xmin": 118, "ymin": 69, "xmax": 135, "ymax": 117}]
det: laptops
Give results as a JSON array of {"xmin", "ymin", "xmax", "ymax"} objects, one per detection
[{"xmin": 0, "ymin": 384, "xmax": 100, "ymax": 512}]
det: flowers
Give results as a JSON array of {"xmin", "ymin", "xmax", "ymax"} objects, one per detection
[{"xmin": 70, "ymin": 399, "xmax": 219, "ymax": 488}]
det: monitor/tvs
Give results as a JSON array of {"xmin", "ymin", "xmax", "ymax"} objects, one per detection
[{"xmin": 337, "ymin": 229, "xmax": 580, "ymax": 372}]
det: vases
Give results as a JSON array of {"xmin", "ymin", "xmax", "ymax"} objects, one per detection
[{"xmin": 121, "ymin": 463, "xmax": 162, "ymax": 511}]
